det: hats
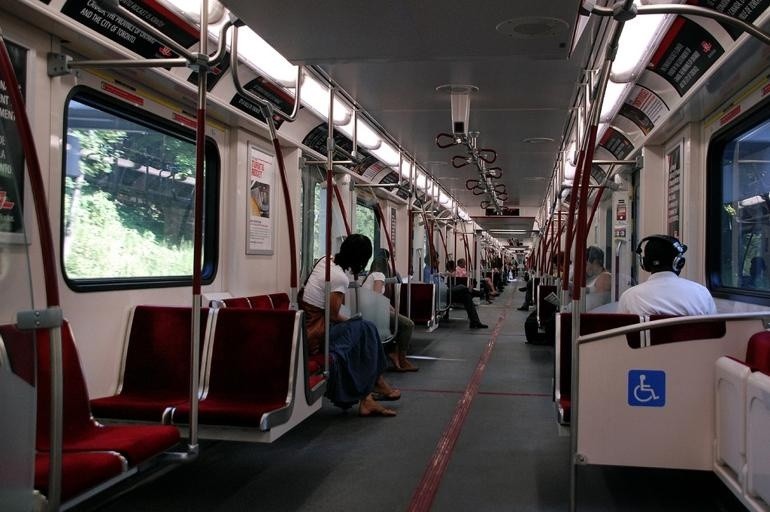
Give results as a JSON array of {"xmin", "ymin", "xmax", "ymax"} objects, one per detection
[{"xmin": 644, "ymin": 235, "xmax": 688, "ymax": 262}]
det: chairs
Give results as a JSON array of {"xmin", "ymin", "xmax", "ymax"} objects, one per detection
[
  {"xmin": 646, "ymin": 315, "xmax": 725, "ymax": 345},
  {"xmin": 447, "ymin": 278, "xmax": 453, "ymax": 284},
  {"xmin": 274, "ymin": 297, "xmax": 341, "ymax": 411},
  {"xmin": 532, "ymin": 277, "xmax": 540, "ymax": 304},
  {"xmin": 705, "ymin": 329, "xmax": 770, "ymax": 510},
  {"xmin": 116, "ymin": 298, "xmax": 213, "ymax": 429},
  {"xmin": 2, "ymin": 328, "xmax": 130, "ymax": 506},
  {"xmin": 536, "ymin": 284, "xmax": 556, "ymax": 326},
  {"xmin": 253, "ymin": 294, "xmax": 268, "ymax": 315},
  {"xmin": 17, "ymin": 324, "xmax": 188, "ymax": 479},
  {"xmin": 176, "ymin": 315, "xmax": 290, "ymax": 449},
  {"xmin": 366, "ymin": 282, "xmax": 400, "ymax": 324},
  {"xmin": 217, "ymin": 297, "xmax": 248, "ymax": 315},
  {"xmin": 400, "ymin": 284, "xmax": 437, "ymax": 335},
  {"xmin": 455, "ymin": 277, "xmax": 468, "ymax": 286},
  {"xmin": 482, "ymin": 271, "xmax": 492, "ymax": 281},
  {"xmin": 746, "ymin": 366, "xmax": 770, "ymax": 508},
  {"xmin": 561, "ymin": 316, "xmax": 637, "ymax": 429}
]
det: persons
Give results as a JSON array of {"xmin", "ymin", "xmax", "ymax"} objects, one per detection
[
  {"xmin": 553, "ymin": 252, "xmax": 575, "ymax": 280},
  {"xmin": 616, "ymin": 233, "xmax": 718, "ymax": 316},
  {"xmin": 360, "ymin": 257, "xmax": 419, "ymax": 371},
  {"xmin": 517, "ymin": 278, "xmax": 540, "ymax": 310},
  {"xmin": 375, "ymin": 248, "xmax": 403, "ymax": 283},
  {"xmin": 303, "ymin": 234, "xmax": 402, "ymax": 417},
  {"xmin": 510, "ymin": 257, "xmax": 518, "ymax": 279},
  {"xmin": 423, "ymin": 252, "xmax": 510, "ymax": 328},
  {"xmin": 750, "ymin": 256, "xmax": 767, "ymax": 279},
  {"xmin": 561, "ymin": 246, "xmax": 611, "ymax": 312}
]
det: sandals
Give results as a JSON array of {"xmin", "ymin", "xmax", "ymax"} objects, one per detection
[
  {"xmin": 387, "ymin": 352, "xmax": 399, "ymax": 369},
  {"xmin": 360, "ymin": 406, "xmax": 397, "ymax": 418},
  {"xmin": 389, "ymin": 360, "xmax": 420, "ymax": 372},
  {"xmin": 371, "ymin": 388, "xmax": 401, "ymax": 401}
]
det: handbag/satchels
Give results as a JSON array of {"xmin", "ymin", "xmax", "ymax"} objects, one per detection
[{"xmin": 296, "ymin": 290, "xmax": 328, "ymax": 354}]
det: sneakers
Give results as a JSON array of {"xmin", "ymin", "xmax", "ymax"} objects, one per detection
[
  {"xmin": 473, "ymin": 290, "xmax": 485, "ymax": 298},
  {"xmin": 519, "ymin": 286, "xmax": 527, "ymax": 292},
  {"xmin": 516, "ymin": 305, "xmax": 530, "ymax": 312},
  {"xmin": 468, "ymin": 321, "xmax": 488, "ymax": 329}
]
